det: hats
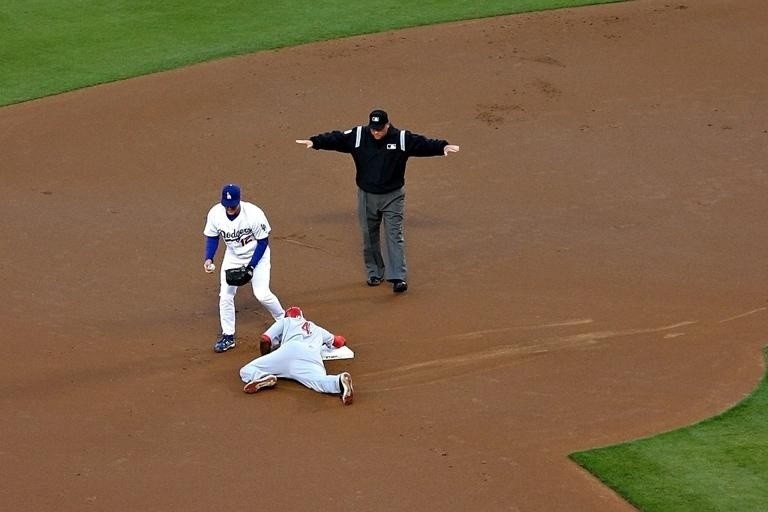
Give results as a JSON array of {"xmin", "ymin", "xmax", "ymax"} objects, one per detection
[
  {"xmin": 366, "ymin": 109, "xmax": 388, "ymax": 130},
  {"xmin": 283, "ymin": 306, "xmax": 303, "ymax": 319},
  {"xmin": 220, "ymin": 183, "xmax": 241, "ymax": 208}
]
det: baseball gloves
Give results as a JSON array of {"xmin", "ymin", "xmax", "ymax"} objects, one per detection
[{"xmin": 225, "ymin": 266, "xmax": 254, "ymax": 286}]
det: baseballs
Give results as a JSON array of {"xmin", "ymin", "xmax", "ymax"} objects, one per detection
[{"xmin": 208, "ymin": 264, "xmax": 215, "ymax": 271}]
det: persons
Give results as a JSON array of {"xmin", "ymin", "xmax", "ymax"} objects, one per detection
[
  {"xmin": 293, "ymin": 108, "xmax": 461, "ymax": 294},
  {"xmin": 202, "ymin": 182, "xmax": 284, "ymax": 353},
  {"xmin": 238, "ymin": 307, "xmax": 355, "ymax": 407}
]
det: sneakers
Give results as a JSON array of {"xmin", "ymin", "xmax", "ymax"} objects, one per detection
[
  {"xmin": 212, "ymin": 336, "xmax": 236, "ymax": 353},
  {"xmin": 337, "ymin": 372, "xmax": 355, "ymax": 405},
  {"xmin": 243, "ymin": 374, "xmax": 278, "ymax": 395},
  {"xmin": 367, "ymin": 276, "xmax": 383, "ymax": 287},
  {"xmin": 393, "ymin": 280, "xmax": 407, "ymax": 294}
]
